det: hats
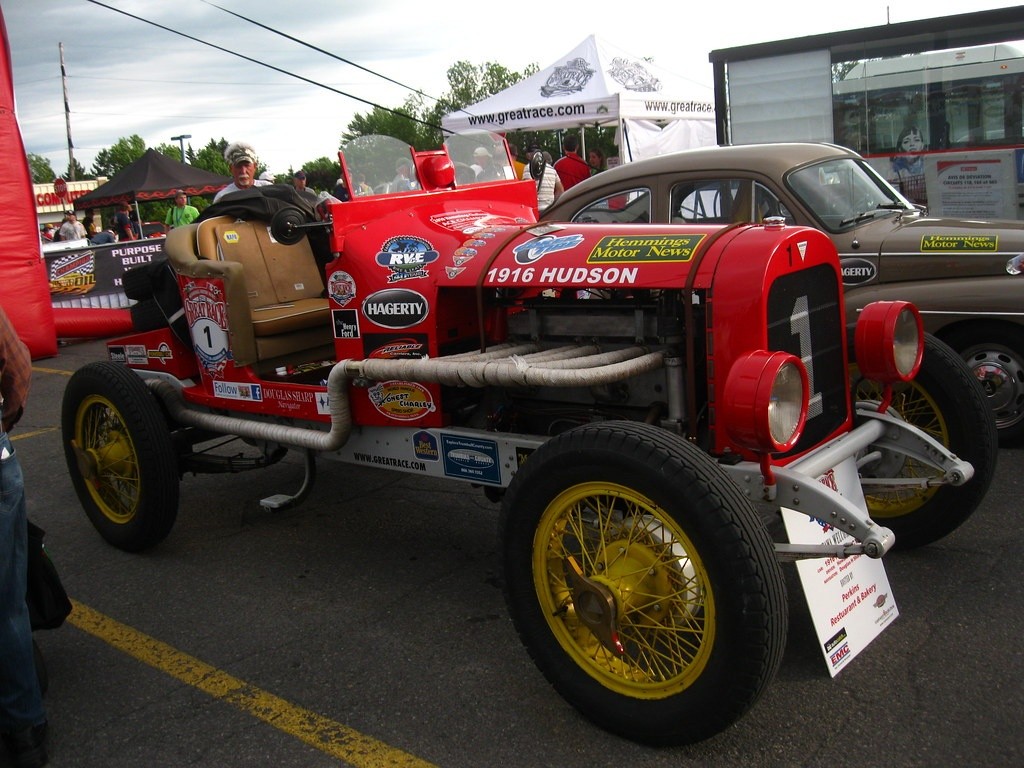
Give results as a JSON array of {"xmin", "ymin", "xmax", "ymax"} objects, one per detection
[
  {"xmin": 563, "ymin": 134, "xmax": 578, "ymax": 146},
  {"xmin": 338, "ymin": 165, "xmax": 352, "ymax": 177},
  {"xmin": 473, "ymin": 146, "xmax": 492, "ymax": 158},
  {"xmin": 64, "ymin": 210, "xmax": 74, "ymax": 215},
  {"xmin": 45, "ymin": 224, "xmax": 54, "ymax": 229},
  {"xmin": 227, "ymin": 145, "xmax": 257, "ymax": 169},
  {"xmin": 526, "ymin": 142, "xmax": 541, "ymax": 152},
  {"xmin": 294, "ymin": 171, "xmax": 306, "ymax": 179},
  {"xmin": 120, "ymin": 201, "xmax": 129, "ymax": 210},
  {"xmin": 174, "ymin": 190, "xmax": 187, "ymax": 199}
]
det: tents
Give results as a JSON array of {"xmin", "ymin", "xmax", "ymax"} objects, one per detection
[
  {"xmin": 441, "ymin": 31, "xmax": 731, "ymax": 218},
  {"xmin": 72, "ymin": 147, "xmax": 235, "ymax": 239}
]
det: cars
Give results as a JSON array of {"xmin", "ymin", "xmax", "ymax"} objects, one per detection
[
  {"xmin": 137, "ymin": 221, "xmax": 170, "ymax": 236},
  {"xmin": 61, "ymin": 133, "xmax": 997, "ymax": 747},
  {"xmin": 536, "ymin": 140, "xmax": 1024, "ymax": 448}
]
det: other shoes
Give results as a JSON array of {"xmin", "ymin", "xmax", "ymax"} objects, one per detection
[{"xmin": 0, "ymin": 711, "xmax": 50, "ymax": 768}]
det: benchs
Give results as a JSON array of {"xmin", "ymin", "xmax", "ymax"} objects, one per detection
[{"xmin": 198, "ymin": 215, "xmax": 332, "ymax": 362}]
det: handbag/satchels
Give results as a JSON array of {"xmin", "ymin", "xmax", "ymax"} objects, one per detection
[{"xmin": 24, "ymin": 520, "xmax": 72, "ymax": 630}]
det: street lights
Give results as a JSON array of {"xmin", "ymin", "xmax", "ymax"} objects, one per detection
[{"xmin": 170, "ymin": 135, "xmax": 191, "ymax": 206}]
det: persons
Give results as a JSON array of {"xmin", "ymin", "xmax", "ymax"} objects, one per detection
[
  {"xmin": 0, "ymin": 305, "xmax": 48, "ymax": 768},
  {"xmin": 332, "ymin": 157, "xmax": 411, "ymax": 202},
  {"xmin": 42, "ymin": 201, "xmax": 141, "ymax": 246},
  {"xmin": 213, "ymin": 140, "xmax": 274, "ymax": 205},
  {"xmin": 259, "ymin": 171, "xmax": 277, "ymax": 184},
  {"xmin": 293, "ymin": 170, "xmax": 317, "ymax": 197},
  {"xmin": 552, "ymin": 134, "xmax": 591, "ymax": 192},
  {"xmin": 522, "ymin": 142, "xmax": 564, "ymax": 215},
  {"xmin": 164, "ymin": 189, "xmax": 200, "ymax": 234},
  {"xmin": 588, "ymin": 147, "xmax": 608, "ymax": 177},
  {"xmin": 895, "ymin": 126, "xmax": 924, "ymax": 152},
  {"xmin": 469, "ymin": 143, "xmax": 553, "ymax": 179}
]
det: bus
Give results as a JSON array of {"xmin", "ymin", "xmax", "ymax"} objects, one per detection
[{"xmin": 834, "ymin": 44, "xmax": 1024, "ymax": 217}]
